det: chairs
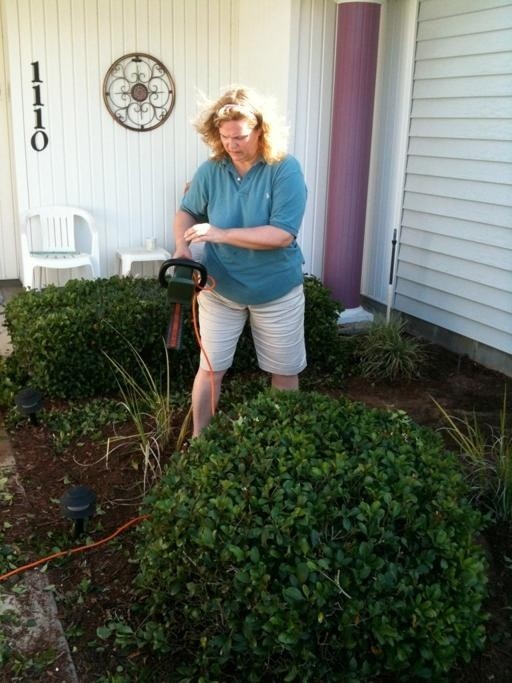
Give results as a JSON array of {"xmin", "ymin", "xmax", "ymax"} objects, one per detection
[{"xmin": 20, "ymin": 203, "xmax": 101, "ymax": 291}]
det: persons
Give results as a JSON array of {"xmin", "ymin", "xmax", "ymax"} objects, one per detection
[{"xmin": 172, "ymin": 90, "xmax": 307, "ymax": 442}]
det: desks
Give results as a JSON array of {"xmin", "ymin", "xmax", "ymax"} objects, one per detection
[{"xmin": 116, "ymin": 240, "xmax": 172, "ymax": 279}]
